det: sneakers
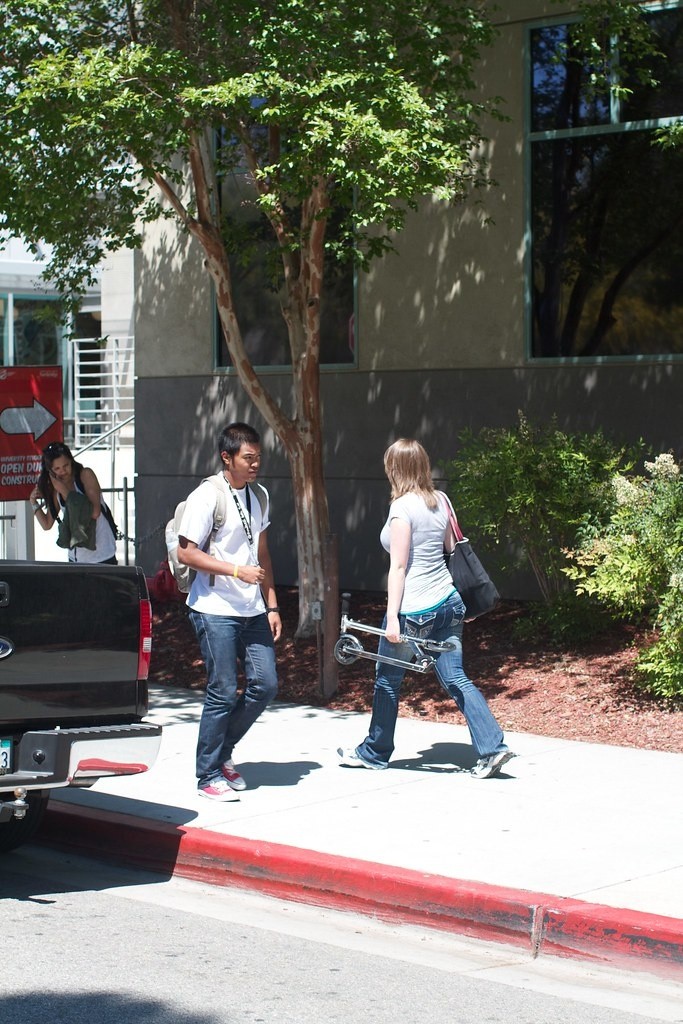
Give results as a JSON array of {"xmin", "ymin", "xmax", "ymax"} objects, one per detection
[
  {"xmin": 470, "ymin": 751, "xmax": 512, "ymax": 778},
  {"xmin": 198, "ymin": 776, "xmax": 240, "ymax": 803},
  {"xmin": 339, "ymin": 750, "xmax": 384, "ymax": 770},
  {"xmin": 221, "ymin": 758, "xmax": 246, "ymax": 791}
]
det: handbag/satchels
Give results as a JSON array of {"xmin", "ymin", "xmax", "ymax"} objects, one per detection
[{"xmin": 437, "ymin": 490, "xmax": 499, "ymax": 622}]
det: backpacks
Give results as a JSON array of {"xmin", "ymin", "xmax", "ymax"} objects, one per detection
[{"xmin": 163, "ymin": 474, "xmax": 268, "ymax": 594}]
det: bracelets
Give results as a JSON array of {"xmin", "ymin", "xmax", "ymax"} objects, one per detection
[
  {"xmin": 233, "ymin": 565, "xmax": 239, "ymax": 577},
  {"xmin": 32, "ymin": 504, "xmax": 40, "ymax": 513},
  {"xmin": 266, "ymin": 608, "xmax": 280, "ymax": 613}
]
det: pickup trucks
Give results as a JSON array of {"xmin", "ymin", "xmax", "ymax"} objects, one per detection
[{"xmin": 0, "ymin": 557, "xmax": 164, "ymax": 855}]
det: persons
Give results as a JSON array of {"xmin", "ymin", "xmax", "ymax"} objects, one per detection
[
  {"xmin": 30, "ymin": 442, "xmax": 118, "ymax": 565},
  {"xmin": 337, "ymin": 439, "xmax": 516, "ymax": 779},
  {"xmin": 179, "ymin": 422, "xmax": 283, "ymax": 802}
]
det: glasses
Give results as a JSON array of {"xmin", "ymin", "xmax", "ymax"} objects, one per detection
[{"xmin": 47, "ymin": 445, "xmax": 66, "ymax": 452}]
